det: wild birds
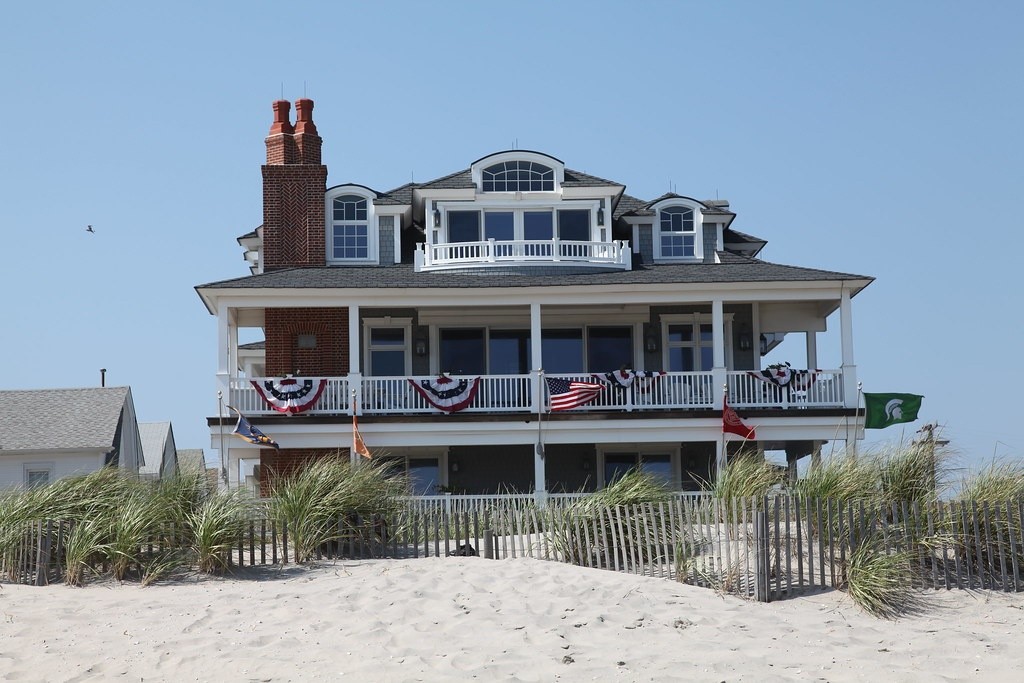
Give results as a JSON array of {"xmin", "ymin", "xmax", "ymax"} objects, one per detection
[{"xmin": 86, "ymin": 224, "xmax": 96, "ymax": 235}]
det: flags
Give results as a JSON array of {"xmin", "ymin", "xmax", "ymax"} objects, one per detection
[
  {"xmin": 545, "ymin": 378, "xmax": 606, "ymax": 411},
  {"xmin": 721, "ymin": 392, "xmax": 754, "ymax": 438},
  {"xmin": 226, "ymin": 405, "xmax": 280, "ymax": 453},
  {"xmin": 862, "ymin": 391, "xmax": 924, "ymax": 430},
  {"xmin": 353, "ymin": 398, "xmax": 372, "ymax": 459}
]
